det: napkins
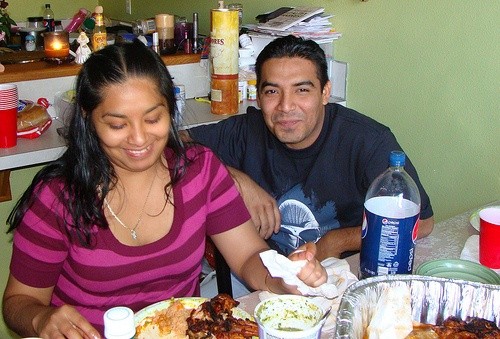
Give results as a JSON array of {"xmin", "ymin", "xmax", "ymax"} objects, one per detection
[{"xmin": 258, "ymin": 249, "xmax": 359, "ymax": 299}]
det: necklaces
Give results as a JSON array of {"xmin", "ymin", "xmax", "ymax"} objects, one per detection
[{"xmin": 98, "ymin": 174, "xmax": 157, "ymax": 240}]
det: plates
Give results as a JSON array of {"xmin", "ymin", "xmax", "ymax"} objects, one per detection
[
  {"xmin": 133, "ymin": 297, "xmax": 260, "ymax": 339},
  {"xmin": 415, "ymin": 259, "xmax": 500, "ymax": 285}
]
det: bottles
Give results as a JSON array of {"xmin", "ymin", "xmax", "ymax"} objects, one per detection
[
  {"xmin": 132, "ymin": 18, "xmax": 157, "ymax": 36},
  {"xmin": 65, "ymin": 7, "xmax": 87, "ymax": 33},
  {"xmin": 238, "ymin": 78, "xmax": 258, "ymax": 104},
  {"xmin": 152, "ymin": 32, "xmax": 161, "ymax": 56},
  {"xmin": 91, "ymin": 5, "xmax": 107, "ymax": 52},
  {"xmin": 13, "ymin": 3, "xmax": 63, "ymax": 51},
  {"xmin": 155, "ymin": 13, "xmax": 174, "ymax": 56},
  {"xmin": 77, "ymin": 18, "xmax": 95, "ymax": 34},
  {"xmin": 174, "ymin": 17, "xmax": 194, "ymax": 50},
  {"xmin": 103, "ymin": 306, "xmax": 137, "ymax": 339},
  {"xmin": 173, "ymin": 84, "xmax": 185, "ymax": 126},
  {"xmin": 357, "ymin": 151, "xmax": 421, "ymax": 281}
]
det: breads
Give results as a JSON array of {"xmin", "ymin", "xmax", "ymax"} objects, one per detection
[{"xmin": 17, "ymin": 100, "xmax": 50, "ymax": 131}]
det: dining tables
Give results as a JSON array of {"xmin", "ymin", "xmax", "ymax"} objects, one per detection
[{"xmin": 231, "ymin": 199, "xmax": 500, "ymax": 339}]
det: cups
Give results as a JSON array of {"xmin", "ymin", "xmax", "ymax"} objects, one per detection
[
  {"xmin": 44, "ymin": 31, "xmax": 69, "ymax": 58},
  {"xmin": 479, "ymin": 207, "xmax": 500, "ymax": 269},
  {"xmin": 229, "ymin": 3, "xmax": 243, "ymax": 26},
  {"xmin": 253, "ymin": 294, "xmax": 324, "ymax": 339},
  {"xmin": 0, "ymin": 84, "xmax": 18, "ymax": 148},
  {"xmin": 184, "ymin": 38, "xmax": 192, "ymax": 53}
]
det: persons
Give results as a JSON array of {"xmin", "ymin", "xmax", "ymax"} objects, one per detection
[
  {"xmin": 178, "ymin": 34, "xmax": 435, "ymax": 299},
  {"xmin": 2, "ymin": 43, "xmax": 328, "ymax": 339}
]
